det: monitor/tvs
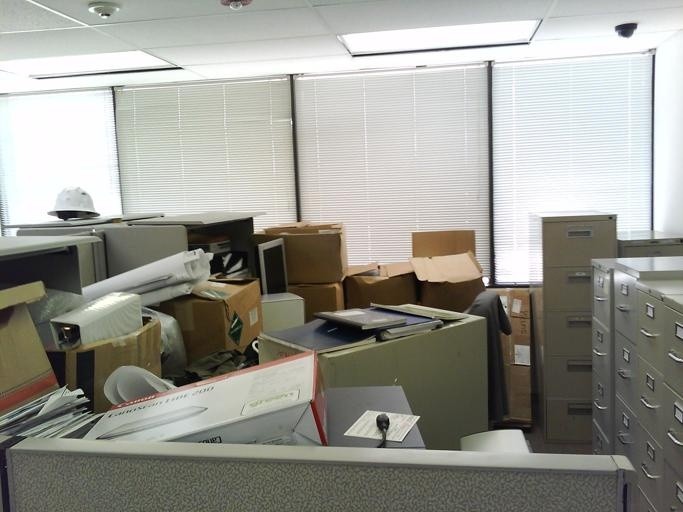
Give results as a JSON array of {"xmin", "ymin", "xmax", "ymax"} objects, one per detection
[{"xmin": 258, "ymin": 237, "xmax": 290, "ymax": 295}]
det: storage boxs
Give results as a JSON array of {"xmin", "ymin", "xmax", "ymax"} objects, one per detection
[{"xmin": 1, "ymin": 221, "xmax": 533, "ymax": 448}]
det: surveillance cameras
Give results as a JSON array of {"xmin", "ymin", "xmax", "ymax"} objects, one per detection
[{"xmin": 615, "ymin": 22, "xmax": 638, "ymax": 39}]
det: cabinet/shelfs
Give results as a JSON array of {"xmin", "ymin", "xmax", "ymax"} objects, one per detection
[
  {"xmin": 590, "ymin": 256, "xmax": 681, "ymax": 497},
  {"xmin": 539, "ymin": 212, "xmax": 618, "ymax": 443}
]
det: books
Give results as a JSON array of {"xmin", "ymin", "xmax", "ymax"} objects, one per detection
[
  {"xmin": 259, "ymin": 303, "xmax": 468, "ymax": 355},
  {"xmin": 127, "ymin": 213, "xmax": 210, "ymax": 224}
]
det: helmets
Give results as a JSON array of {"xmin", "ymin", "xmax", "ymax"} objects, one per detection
[{"xmin": 47, "ymin": 187, "xmax": 100, "ymax": 219}]
